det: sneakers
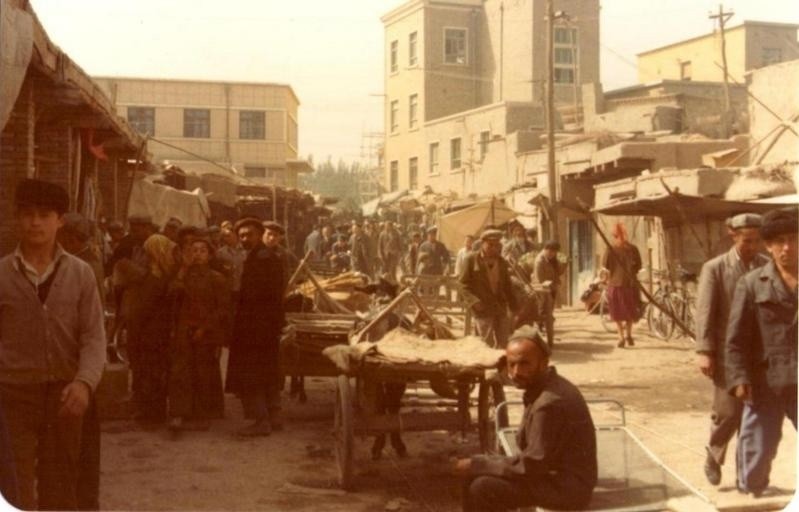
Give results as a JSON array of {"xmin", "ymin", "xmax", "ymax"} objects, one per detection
[{"xmin": 135, "ymin": 410, "xmax": 282, "ymax": 437}]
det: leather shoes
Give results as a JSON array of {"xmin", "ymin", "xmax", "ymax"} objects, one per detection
[
  {"xmin": 618, "ymin": 337, "xmax": 633, "ymax": 347},
  {"xmin": 706, "ymin": 450, "xmax": 721, "ymax": 483}
]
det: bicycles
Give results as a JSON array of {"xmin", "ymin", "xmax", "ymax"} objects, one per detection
[{"xmin": 597, "ymin": 264, "xmax": 698, "ymax": 341}]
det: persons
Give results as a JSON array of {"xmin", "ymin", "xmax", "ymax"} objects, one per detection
[
  {"xmin": 450, "ymin": 323, "xmax": 598, "ymax": 510},
  {"xmin": 0, "ymin": 180, "xmax": 295, "ymax": 511},
  {"xmin": 602, "ymin": 224, "xmax": 642, "ymax": 347},
  {"xmin": 299, "ymin": 207, "xmax": 564, "ymax": 351},
  {"xmin": 694, "ymin": 209, "xmax": 798, "ymax": 511}
]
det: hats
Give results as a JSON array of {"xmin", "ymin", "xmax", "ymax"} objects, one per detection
[
  {"xmin": 70, "ymin": 214, "xmax": 281, "ymax": 253},
  {"xmin": 509, "ymin": 325, "xmax": 552, "ymax": 358},
  {"xmin": 480, "ymin": 229, "xmax": 501, "ymax": 241},
  {"xmin": 543, "ymin": 242, "xmax": 558, "ymax": 248},
  {"xmin": 15, "ymin": 182, "xmax": 69, "ymax": 214},
  {"xmin": 726, "ymin": 211, "xmax": 799, "ymax": 240}
]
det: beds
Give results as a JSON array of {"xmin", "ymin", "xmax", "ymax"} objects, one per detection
[{"xmin": 494, "ymin": 399, "xmax": 720, "ymax": 512}]
